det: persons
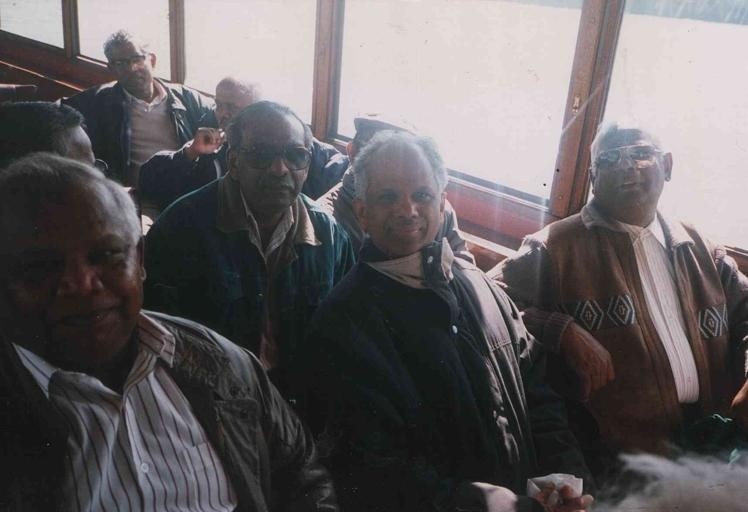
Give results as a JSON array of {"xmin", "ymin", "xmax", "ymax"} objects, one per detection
[
  {"xmin": 0, "ymin": 150, "xmax": 342, "ymax": 512},
  {"xmin": 618, "ymin": 450, "xmax": 748, "ymax": 512},
  {"xmin": 136, "ymin": 77, "xmax": 348, "ymax": 202},
  {"xmin": 313, "ymin": 113, "xmax": 476, "ymax": 267},
  {"xmin": 0, "ymin": 100, "xmax": 164, "ymax": 236},
  {"xmin": 485, "ymin": 121, "xmax": 748, "ymax": 463},
  {"xmin": 300, "ymin": 129, "xmax": 617, "ymax": 512},
  {"xmin": 141, "ymin": 99, "xmax": 356, "ymax": 414},
  {"xmin": 59, "ymin": 29, "xmax": 215, "ymax": 187}
]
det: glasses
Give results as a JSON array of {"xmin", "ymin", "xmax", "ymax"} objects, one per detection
[
  {"xmin": 112, "ymin": 51, "xmax": 150, "ymax": 71},
  {"xmin": 93, "ymin": 159, "xmax": 109, "ymax": 175},
  {"xmin": 592, "ymin": 144, "xmax": 662, "ymax": 172},
  {"xmin": 237, "ymin": 145, "xmax": 313, "ymax": 170}
]
